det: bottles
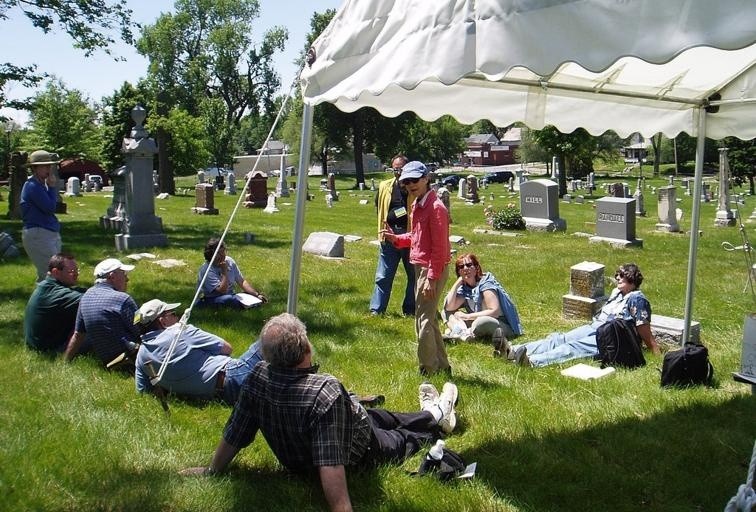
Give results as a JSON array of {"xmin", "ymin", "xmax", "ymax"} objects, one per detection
[{"xmin": 120, "ymin": 337, "xmax": 136, "ymax": 352}]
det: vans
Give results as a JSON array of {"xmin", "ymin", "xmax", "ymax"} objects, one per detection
[{"xmin": 482, "ymin": 169, "xmax": 514, "ymax": 185}]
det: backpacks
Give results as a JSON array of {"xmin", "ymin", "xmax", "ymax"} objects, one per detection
[{"xmin": 596, "ymin": 318, "xmax": 646, "ymax": 371}]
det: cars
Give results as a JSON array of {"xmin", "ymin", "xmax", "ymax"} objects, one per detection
[{"xmin": 425, "ymin": 162, "xmax": 438, "ymax": 172}]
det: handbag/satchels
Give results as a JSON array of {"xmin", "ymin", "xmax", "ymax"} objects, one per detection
[
  {"xmin": 661, "ymin": 345, "xmax": 708, "ymax": 388},
  {"xmin": 419, "ymin": 448, "xmax": 466, "ymax": 481}
]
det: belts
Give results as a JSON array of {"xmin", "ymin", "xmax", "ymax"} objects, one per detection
[{"xmin": 214, "ymin": 365, "xmax": 226, "ymax": 402}]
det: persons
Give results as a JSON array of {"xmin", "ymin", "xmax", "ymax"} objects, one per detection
[
  {"xmin": 64, "ymin": 258, "xmax": 140, "ymax": 378},
  {"xmin": 133, "ymin": 299, "xmax": 266, "ymax": 404},
  {"xmin": 177, "ymin": 313, "xmax": 458, "ymax": 512},
  {"xmin": 25, "ymin": 253, "xmax": 94, "ymax": 362},
  {"xmin": 20, "ymin": 150, "xmax": 62, "ymax": 285},
  {"xmin": 376, "ymin": 161, "xmax": 451, "ymax": 378},
  {"xmin": 369, "ymin": 156, "xmax": 416, "ymax": 317},
  {"xmin": 442, "ymin": 254, "xmax": 525, "ymax": 345},
  {"xmin": 492, "ymin": 264, "xmax": 661, "ymax": 367},
  {"xmin": 198, "ymin": 238, "xmax": 268, "ymax": 311}
]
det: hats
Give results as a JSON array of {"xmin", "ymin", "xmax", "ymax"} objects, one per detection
[
  {"xmin": 398, "ymin": 161, "xmax": 429, "ymax": 182},
  {"xmin": 94, "ymin": 258, "xmax": 136, "ymax": 279},
  {"xmin": 139, "ymin": 298, "xmax": 181, "ymax": 328},
  {"xmin": 25, "ymin": 149, "xmax": 57, "ymax": 168}
]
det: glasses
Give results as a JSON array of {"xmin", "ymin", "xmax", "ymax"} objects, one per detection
[
  {"xmin": 402, "ymin": 175, "xmax": 425, "ymax": 185},
  {"xmin": 108, "ymin": 270, "xmax": 128, "ymax": 277},
  {"xmin": 615, "ymin": 271, "xmax": 628, "ymax": 279},
  {"xmin": 157, "ymin": 309, "xmax": 177, "ymax": 322},
  {"xmin": 458, "ymin": 263, "xmax": 473, "ymax": 269},
  {"xmin": 62, "ymin": 266, "xmax": 80, "ymax": 277}
]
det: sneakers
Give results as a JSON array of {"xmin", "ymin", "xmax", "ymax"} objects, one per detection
[
  {"xmin": 257, "ymin": 295, "xmax": 269, "ymax": 304},
  {"xmin": 356, "ymin": 395, "xmax": 385, "ymax": 407},
  {"xmin": 492, "ymin": 328, "xmax": 514, "ymax": 360},
  {"xmin": 433, "ymin": 382, "xmax": 458, "ymax": 433},
  {"xmin": 418, "ymin": 382, "xmax": 439, "ymax": 411},
  {"xmin": 443, "ymin": 330, "xmax": 476, "ymax": 343},
  {"xmin": 513, "ymin": 345, "xmax": 533, "ymax": 368},
  {"xmin": 420, "ymin": 365, "xmax": 452, "ymax": 379}
]
